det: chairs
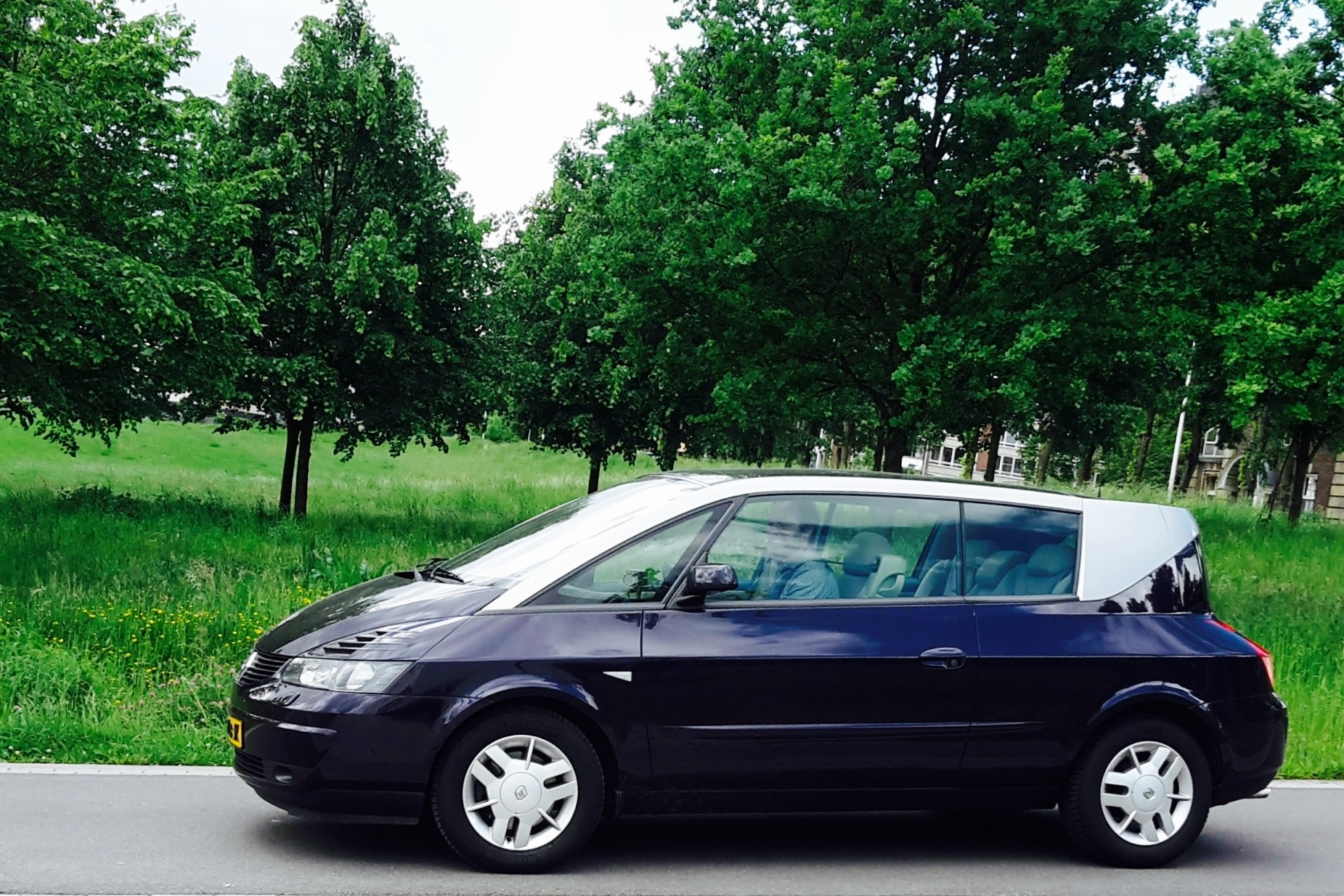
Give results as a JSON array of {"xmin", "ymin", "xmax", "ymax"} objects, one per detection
[{"xmin": 832, "ymin": 532, "xmax": 1077, "ymax": 599}]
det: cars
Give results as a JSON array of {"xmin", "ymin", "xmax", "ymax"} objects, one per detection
[{"xmin": 226, "ymin": 469, "xmax": 1289, "ymax": 876}]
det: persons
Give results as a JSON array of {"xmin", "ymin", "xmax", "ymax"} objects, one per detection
[{"xmin": 720, "ymin": 499, "xmax": 840, "ymax": 601}]
